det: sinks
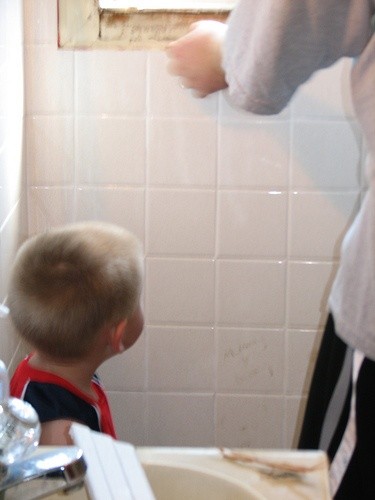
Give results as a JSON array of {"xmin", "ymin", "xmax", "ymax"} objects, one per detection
[{"xmin": 0, "ymin": 445, "xmax": 332, "ymax": 500}]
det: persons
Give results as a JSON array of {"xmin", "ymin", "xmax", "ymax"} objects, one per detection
[
  {"xmin": 166, "ymin": 0, "xmax": 375, "ymax": 500},
  {"xmin": 9, "ymin": 224, "xmax": 144, "ymax": 447}
]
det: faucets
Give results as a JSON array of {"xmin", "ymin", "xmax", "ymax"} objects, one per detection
[{"xmin": 0, "ymin": 444, "xmax": 88, "ymax": 500}]
